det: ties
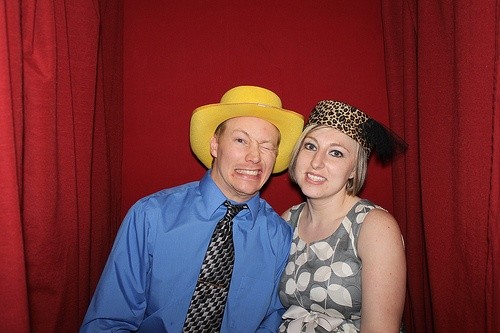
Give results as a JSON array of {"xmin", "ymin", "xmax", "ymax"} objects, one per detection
[{"xmin": 182, "ymin": 200, "xmax": 248, "ymax": 333}]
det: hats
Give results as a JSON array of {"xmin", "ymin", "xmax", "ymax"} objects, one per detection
[
  {"xmin": 190, "ymin": 86, "xmax": 304, "ymax": 174},
  {"xmin": 303, "ymin": 100, "xmax": 408, "ymax": 167}
]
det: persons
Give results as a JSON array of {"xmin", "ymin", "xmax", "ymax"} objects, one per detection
[
  {"xmin": 280, "ymin": 99, "xmax": 407, "ymax": 333},
  {"xmin": 80, "ymin": 85, "xmax": 305, "ymax": 333}
]
